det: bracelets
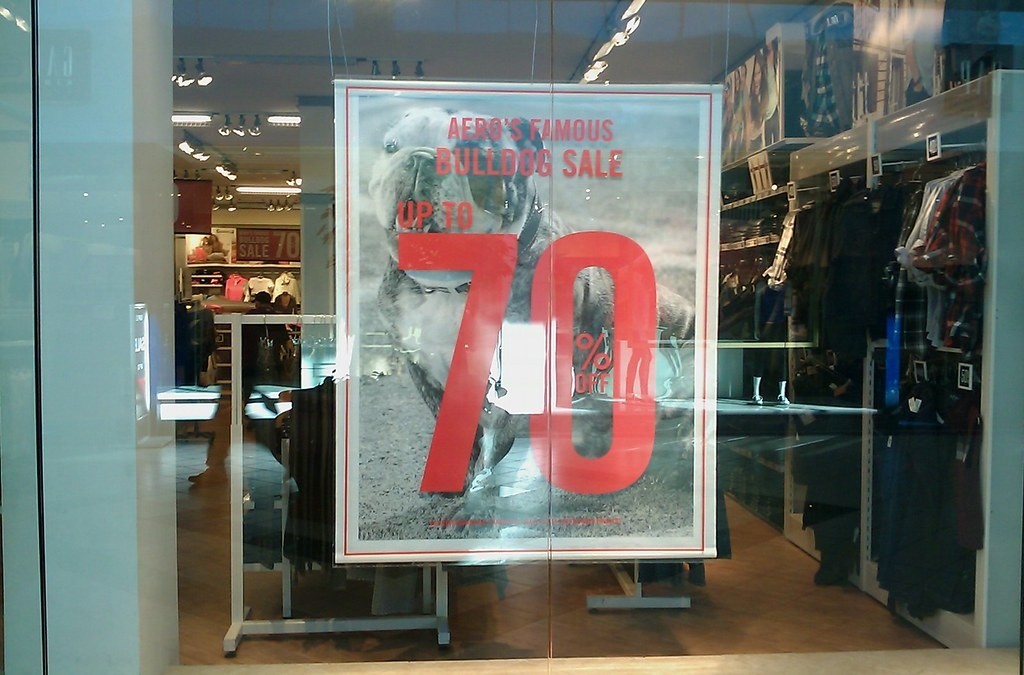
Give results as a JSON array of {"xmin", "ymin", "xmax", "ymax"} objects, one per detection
[{"xmin": 913, "ymin": 77, "xmax": 921, "ymax": 86}]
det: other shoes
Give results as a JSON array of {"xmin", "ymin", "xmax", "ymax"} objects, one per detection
[
  {"xmin": 188, "ymin": 466, "xmax": 228, "ymax": 482},
  {"xmin": 778, "ymin": 395, "xmax": 790, "ymax": 405},
  {"xmin": 753, "ymin": 395, "xmax": 763, "ymax": 404}
]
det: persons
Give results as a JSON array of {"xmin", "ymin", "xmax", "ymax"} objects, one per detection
[
  {"xmin": 188, "ymin": 235, "xmax": 229, "ymax": 261},
  {"xmin": 900, "ymin": 0, "xmax": 945, "ymax": 107},
  {"xmin": 721, "ymin": 39, "xmax": 780, "ymax": 164},
  {"xmin": 244, "ymin": 291, "xmax": 292, "ymax": 384},
  {"xmin": 275, "ymin": 292, "xmax": 296, "ymax": 313},
  {"xmin": 186, "ymin": 296, "xmax": 215, "ymax": 372},
  {"xmin": 187, "ymin": 315, "xmax": 258, "ymax": 481}
]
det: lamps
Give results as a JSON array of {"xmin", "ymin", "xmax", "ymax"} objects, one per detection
[
  {"xmin": 286, "ymin": 171, "xmax": 302, "ymax": 187},
  {"xmin": 172, "ymin": 111, "xmax": 212, "ymax": 127},
  {"xmin": 212, "ymin": 186, "xmax": 236, "ymax": 212},
  {"xmin": 218, "ymin": 113, "xmax": 263, "ymax": 138},
  {"xmin": 265, "ymin": 199, "xmax": 293, "ymax": 212},
  {"xmin": 215, "ymin": 159, "xmax": 238, "ymax": 181},
  {"xmin": 236, "ymin": 188, "xmax": 303, "ymax": 198},
  {"xmin": 170, "ymin": 55, "xmax": 212, "ymax": 87},
  {"xmin": 267, "ymin": 113, "xmax": 301, "ymax": 128},
  {"xmin": 177, "ymin": 130, "xmax": 210, "ymax": 162},
  {"xmin": 567, "ymin": 0, "xmax": 647, "ymax": 84}
]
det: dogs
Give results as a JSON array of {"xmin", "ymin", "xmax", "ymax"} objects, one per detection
[{"xmin": 370, "ymin": 108, "xmax": 694, "ymax": 491}]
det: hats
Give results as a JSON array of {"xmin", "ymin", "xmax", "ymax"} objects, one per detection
[{"xmin": 251, "ymin": 292, "xmax": 271, "ymax": 304}]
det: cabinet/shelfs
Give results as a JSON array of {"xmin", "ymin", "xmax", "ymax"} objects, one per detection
[{"xmin": 188, "ymin": 264, "xmax": 301, "ymax": 287}]
